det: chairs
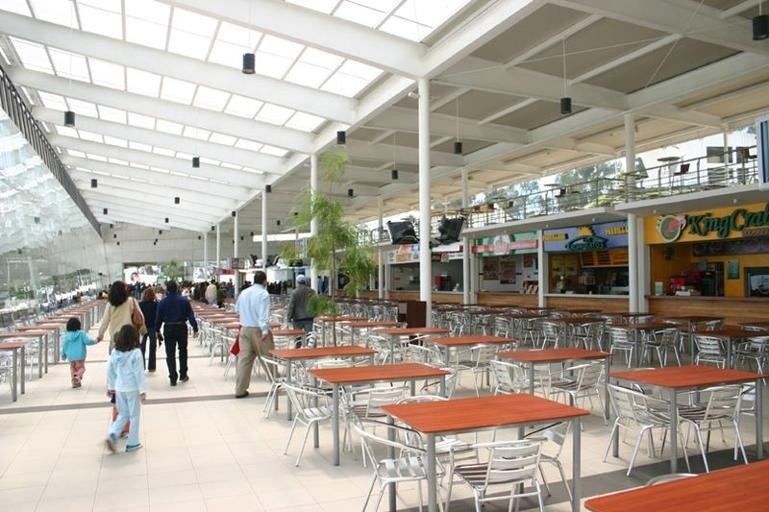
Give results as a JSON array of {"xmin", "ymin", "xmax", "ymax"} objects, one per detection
[
  {"xmin": 0, "ymin": 297, "xmax": 107, "ymax": 402},
  {"xmin": 363, "ymin": 140, "xmax": 766, "ymax": 224},
  {"xmin": 307, "ymin": 361, "xmax": 452, "ymax": 466},
  {"xmin": 393, "ymin": 393, "xmax": 482, "ymax": 511},
  {"xmin": 605, "ymin": 363, "xmax": 768, "ymax": 477},
  {"xmin": 278, "ymin": 379, "xmax": 368, "ymax": 468},
  {"xmin": 507, "ymin": 391, "xmax": 573, "ymax": 512},
  {"xmin": 443, "ymin": 437, "xmax": 544, "ymax": 511},
  {"xmin": 186, "ymin": 293, "xmax": 769, "ymax": 431},
  {"xmin": 352, "ymin": 418, "xmax": 448, "ymax": 511}
]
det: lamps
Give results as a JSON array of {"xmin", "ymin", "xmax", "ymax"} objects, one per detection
[
  {"xmin": 454, "ymin": 95, "xmax": 461, "ymax": 153},
  {"xmin": 147, "ymin": 154, "xmax": 201, "ymax": 248},
  {"xmin": 749, "ymin": 0, "xmax": 769, "ymax": 40},
  {"xmin": 558, "ymin": 35, "xmax": 571, "ymax": 114},
  {"xmin": 65, "ymin": 109, "xmax": 122, "ymax": 248},
  {"xmin": 241, "ymin": 0, "xmax": 257, "ymax": 75},
  {"xmin": 205, "ymin": 127, "xmax": 399, "ymax": 242}
]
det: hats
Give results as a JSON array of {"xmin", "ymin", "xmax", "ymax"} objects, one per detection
[{"xmin": 296, "ymin": 274, "xmax": 306, "ymax": 284}]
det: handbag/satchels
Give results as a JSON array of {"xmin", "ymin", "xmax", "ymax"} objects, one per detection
[{"xmin": 132, "ymin": 310, "xmax": 143, "ymax": 327}]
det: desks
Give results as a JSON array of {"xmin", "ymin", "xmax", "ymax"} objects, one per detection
[
  {"xmin": 379, "ymin": 391, "xmax": 593, "ymax": 512},
  {"xmin": 583, "ymin": 458, "xmax": 768, "ymax": 510}
]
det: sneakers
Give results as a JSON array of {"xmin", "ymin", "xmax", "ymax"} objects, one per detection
[
  {"xmin": 105, "ymin": 433, "xmax": 116, "ymax": 452},
  {"xmin": 71, "ymin": 377, "xmax": 82, "ymax": 388},
  {"xmin": 125, "ymin": 444, "xmax": 142, "ymax": 451}
]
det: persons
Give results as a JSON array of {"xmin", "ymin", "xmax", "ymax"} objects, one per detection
[
  {"xmin": 105, "ymin": 324, "xmax": 146, "ymax": 454},
  {"xmin": 60, "ymin": 316, "xmax": 99, "ymax": 388},
  {"xmin": 138, "ymin": 288, "xmax": 158, "ymax": 372},
  {"xmin": 98, "ymin": 281, "xmax": 147, "ymax": 402},
  {"xmin": 287, "ymin": 275, "xmax": 317, "ymax": 348},
  {"xmin": 111, "ymin": 332, "xmax": 129, "ymax": 437},
  {"xmin": 155, "ymin": 281, "xmax": 199, "ymax": 386},
  {"xmin": 235, "ymin": 271, "xmax": 280, "ymax": 398},
  {"xmin": 95, "ymin": 280, "xmax": 286, "ymax": 309},
  {"xmin": 318, "ymin": 274, "xmax": 348, "ymax": 294}
]
